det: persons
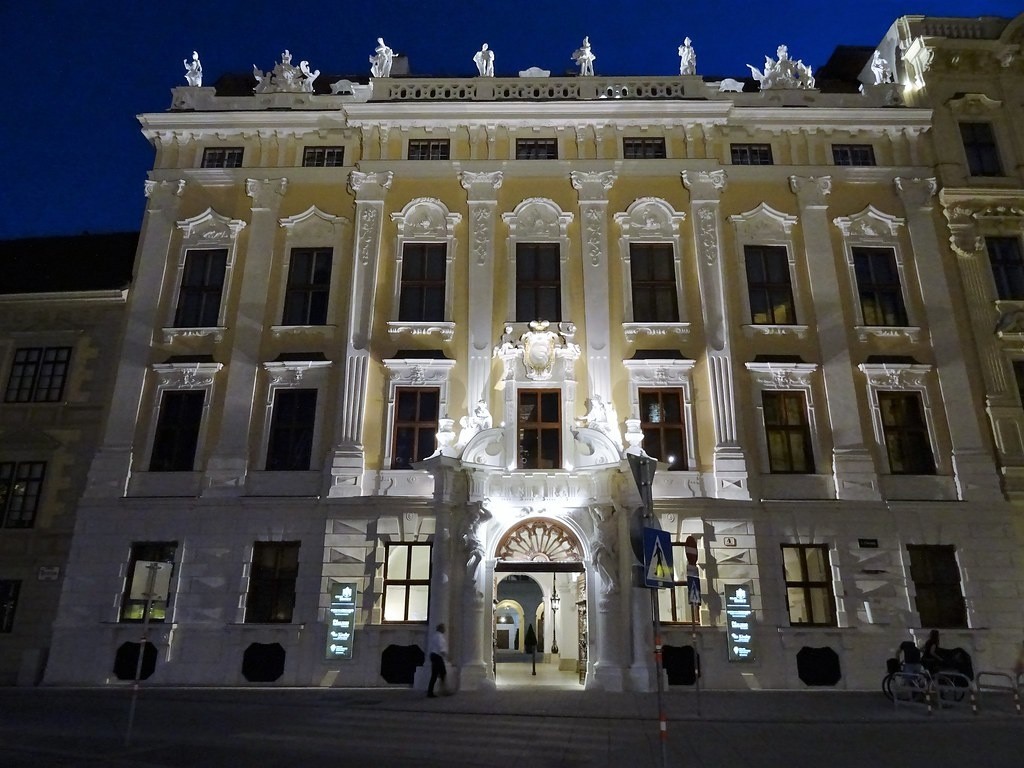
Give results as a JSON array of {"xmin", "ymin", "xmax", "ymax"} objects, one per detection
[
  {"xmin": 252, "ymin": 49, "xmax": 319, "ymax": 93},
  {"xmin": 184, "ymin": 51, "xmax": 203, "ymax": 88},
  {"xmin": 896, "ymin": 641, "xmax": 922, "ymax": 700},
  {"xmin": 427, "ymin": 623, "xmax": 454, "ymax": 697},
  {"xmin": 572, "ymin": 36, "xmax": 597, "ymax": 76},
  {"xmin": 871, "ymin": 50, "xmax": 892, "ymax": 85},
  {"xmin": 474, "ymin": 43, "xmax": 495, "ymax": 76},
  {"xmin": 458, "ymin": 320, "xmax": 625, "ymax": 452},
  {"xmin": 678, "ymin": 36, "xmax": 697, "ymax": 76},
  {"xmin": 745, "ymin": 42, "xmax": 817, "ymax": 91},
  {"xmin": 924, "ymin": 630, "xmax": 950, "ymax": 707},
  {"xmin": 369, "ymin": 38, "xmax": 398, "ymax": 79}
]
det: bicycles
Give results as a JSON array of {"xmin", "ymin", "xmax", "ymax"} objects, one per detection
[{"xmin": 881, "ymin": 658, "xmax": 966, "ymax": 706}]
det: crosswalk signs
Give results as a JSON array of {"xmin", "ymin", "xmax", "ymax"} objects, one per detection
[
  {"xmin": 686, "ymin": 575, "xmax": 701, "ymax": 605},
  {"xmin": 641, "ymin": 527, "xmax": 675, "ymax": 590}
]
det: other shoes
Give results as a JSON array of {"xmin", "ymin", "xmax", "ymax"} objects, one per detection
[
  {"xmin": 444, "ymin": 692, "xmax": 454, "ymax": 696},
  {"xmin": 427, "ymin": 693, "xmax": 436, "ymax": 698}
]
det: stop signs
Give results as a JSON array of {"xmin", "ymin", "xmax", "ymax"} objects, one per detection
[{"xmin": 685, "ymin": 536, "xmax": 698, "ymax": 566}]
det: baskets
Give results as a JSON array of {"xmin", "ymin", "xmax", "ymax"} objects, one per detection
[{"xmin": 886, "ymin": 659, "xmax": 902, "ymax": 673}]
